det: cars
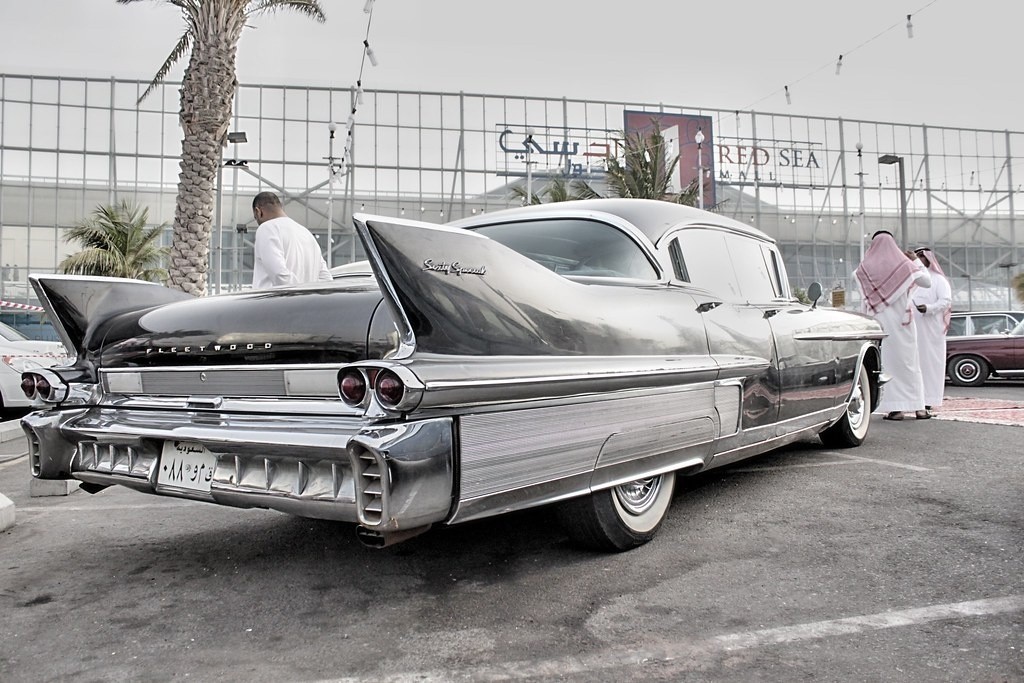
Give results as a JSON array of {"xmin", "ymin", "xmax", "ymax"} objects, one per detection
[
  {"xmin": 19, "ymin": 193, "xmax": 887, "ymax": 555},
  {"xmin": 2, "ymin": 303, "xmax": 82, "ymax": 411}
]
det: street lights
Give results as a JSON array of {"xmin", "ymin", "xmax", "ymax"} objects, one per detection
[
  {"xmin": 692, "ymin": 125, "xmax": 705, "ymax": 210},
  {"xmin": 853, "ymin": 142, "xmax": 866, "ymax": 264},
  {"xmin": 879, "ymin": 153, "xmax": 910, "ymax": 258},
  {"xmin": 527, "ymin": 126, "xmax": 535, "ymax": 208}
]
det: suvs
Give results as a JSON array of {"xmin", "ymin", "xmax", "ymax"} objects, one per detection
[{"xmin": 944, "ymin": 307, "xmax": 1024, "ymax": 385}]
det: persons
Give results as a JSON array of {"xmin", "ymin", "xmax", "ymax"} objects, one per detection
[
  {"xmin": 850, "ymin": 230, "xmax": 932, "ymax": 423},
  {"xmin": 909, "ymin": 247, "xmax": 953, "ymax": 417},
  {"xmin": 250, "ymin": 192, "xmax": 334, "ymax": 292}
]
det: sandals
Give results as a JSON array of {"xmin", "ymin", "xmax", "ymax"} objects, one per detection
[
  {"xmin": 917, "ymin": 411, "xmax": 937, "ymax": 419},
  {"xmin": 883, "ymin": 412, "xmax": 904, "ymax": 420}
]
